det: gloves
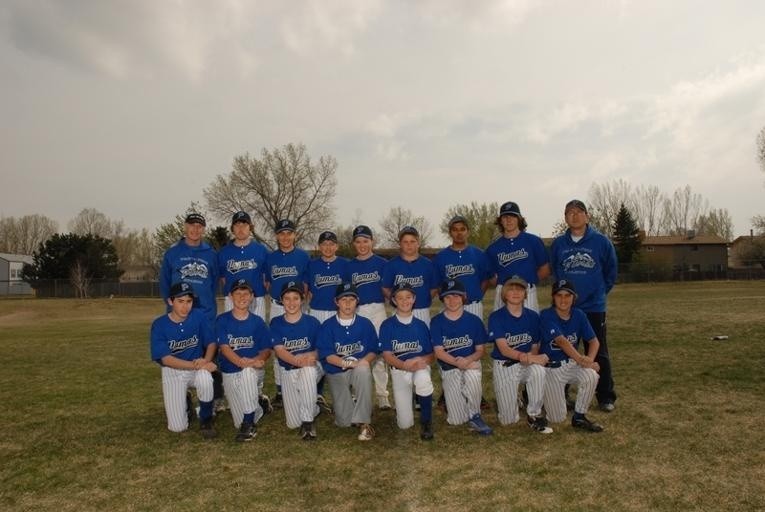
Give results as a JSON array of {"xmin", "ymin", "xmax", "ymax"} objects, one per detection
[{"xmin": 341, "ymin": 355, "xmax": 359, "ymax": 370}]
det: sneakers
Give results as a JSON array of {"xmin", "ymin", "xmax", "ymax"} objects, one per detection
[
  {"xmin": 421, "ymin": 422, "xmax": 436, "ymax": 441},
  {"xmin": 200, "ymin": 398, "xmax": 226, "ymax": 438},
  {"xmin": 571, "ymin": 415, "xmax": 603, "ymax": 433},
  {"xmin": 526, "ymin": 414, "xmax": 554, "ymax": 434},
  {"xmin": 375, "ymin": 395, "xmax": 392, "ymax": 410},
  {"xmin": 235, "ymin": 421, "xmax": 259, "ymax": 442},
  {"xmin": 358, "ymin": 423, "xmax": 376, "ymax": 441},
  {"xmin": 258, "ymin": 391, "xmax": 284, "ymax": 415},
  {"xmin": 438, "ymin": 391, "xmax": 578, "ymax": 410},
  {"xmin": 468, "ymin": 413, "xmax": 493, "ymax": 436},
  {"xmin": 186, "ymin": 389, "xmax": 197, "ymax": 421},
  {"xmin": 300, "ymin": 420, "xmax": 317, "ymax": 439},
  {"xmin": 413, "ymin": 394, "xmax": 421, "ymax": 409},
  {"xmin": 316, "ymin": 393, "xmax": 334, "ymax": 415},
  {"xmin": 600, "ymin": 401, "xmax": 615, "ymax": 412}
]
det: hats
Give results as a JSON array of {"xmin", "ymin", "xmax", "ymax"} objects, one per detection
[
  {"xmin": 275, "ymin": 219, "xmax": 296, "ymax": 234},
  {"xmin": 503, "ymin": 273, "xmax": 529, "ymax": 289},
  {"xmin": 231, "ymin": 211, "xmax": 252, "ymax": 226},
  {"xmin": 230, "ymin": 278, "xmax": 253, "ymax": 293},
  {"xmin": 564, "ymin": 200, "xmax": 588, "ymax": 213},
  {"xmin": 352, "ymin": 225, "xmax": 372, "ymax": 240},
  {"xmin": 169, "ymin": 282, "xmax": 194, "ymax": 298},
  {"xmin": 440, "ymin": 279, "xmax": 467, "ymax": 298},
  {"xmin": 318, "ymin": 231, "xmax": 337, "ymax": 244},
  {"xmin": 391, "ymin": 281, "xmax": 417, "ymax": 297},
  {"xmin": 184, "ymin": 213, "xmax": 206, "ymax": 227},
  {"xmin": 398, "ymin": 225, "xmax": 420, "ymax": 240},
  {"xmin": 447, "ymin": 216, "xmax": 470, "ymax": 229},
  {"xmin": 499, "ymin": 201, "xmax": 521, "ymax": 217},
  {"xmin": 552, "ymin": 279, "xmax": 577, "ymax": 296},
  {"xmin": 280, "ymin": 280, "xmax": 304, "ymax": 297},
  {"xmin": 335, "ymin": 283, "xmax": 359, "ymax": 299}
]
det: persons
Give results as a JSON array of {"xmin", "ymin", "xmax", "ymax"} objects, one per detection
[
  {"xmin": 160, "ymin": 201, "xmax": 546, "ymax": 324},
  {"xmin": 548, "ymin": 199, "xmax": 616, "ymax": 411},
  {"xmin": 149, "ymin": 280, "xmax": 618, "ymax": 442}
]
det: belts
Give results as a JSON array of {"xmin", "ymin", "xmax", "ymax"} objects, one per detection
[{"xmin": 544, "ymin": 357, "xmax": 573, "ymax": 369}]
